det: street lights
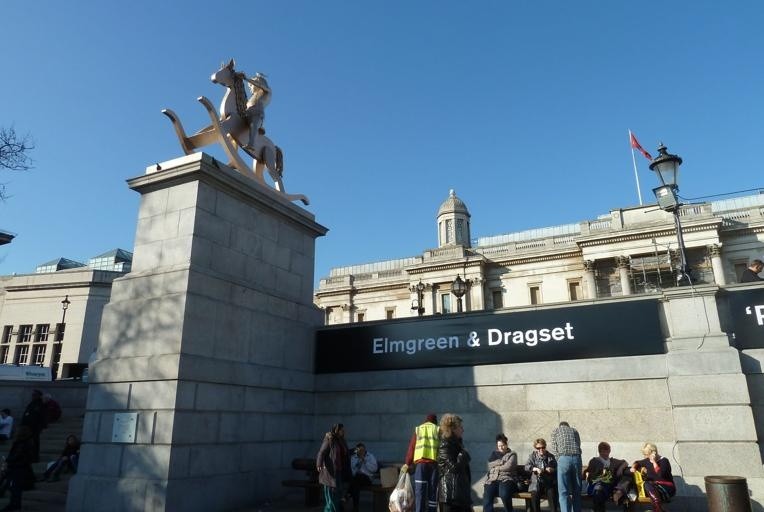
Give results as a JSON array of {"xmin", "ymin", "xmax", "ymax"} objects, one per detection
[
  {"xmin": 650, "ymin": 139, "xmax": 696, "ymax": 286},
  {"xmin": 452, "ymin": 272, "xmax": 468, "ymax": 312},
  {"xmin": 51, "ymin": 294, "xmax": 70, "ymax": 379},
  {"xmin": 412, "ymin": 279, "xmax": 426, "ymax": 316}
]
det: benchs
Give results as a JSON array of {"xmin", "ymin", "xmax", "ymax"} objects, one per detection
[
  {"xmin": 282, "ymin": 456, "xmax": 405, "ymax": 493},
  {"xmin": 497, "ymin": 466, "xmax": 652, "ymax": 512}
]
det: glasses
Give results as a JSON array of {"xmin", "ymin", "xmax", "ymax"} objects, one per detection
[
  {"xmin": 536, "ymin": 447, "xmax": 544, "ymax": 449},
  {"xmin": 559, "ymin": 454, "xmax": 581, "ymax": 457}
]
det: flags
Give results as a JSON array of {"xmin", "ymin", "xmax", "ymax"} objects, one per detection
[{"xmin": 631, "ymin": 133, "xmax": 651, "ymax": 160}]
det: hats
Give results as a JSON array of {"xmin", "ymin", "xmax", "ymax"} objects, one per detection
[{"xmin": 425, "ymin": 414, "xmax": 437, "ymax": 425}]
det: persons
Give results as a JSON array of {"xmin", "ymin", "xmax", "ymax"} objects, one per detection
[
  {"xmin": 481, "ymin": 432, "xmax": 518, "ymax": 511},
  {"xmin": 550, "ymin": 420, "xmax": 584, "ymax": 511},
  {"xmin": 612, "ymin": 441, "xmax": 675, "ymax": 512},
  {"xmin": 524, "ymin": 438, "xmax": 557, "ymax": 511},
  {"xmin": 582, "ymin": 441, "xmax": 628, "ymax": 511},
  {"xmin": 437, "ymin": 413, "xmax": 474, "ymax": 511},
  {"xmin": 741, "ymin": 259, "xmax": 764, "ymax": 283},
  {"xmin": 226, "ymin": 72, "xmax": 273, "ymax": 152},
  {"xmin": 402, "ymin": 413, "xmax": 441, "ymax": 511},
  {"xmin": 0, "ymin": 384, "xmax": 80, "ymax": 511},
  {"xmin": 315, "ymin": 421, "xmax": 344, "ymax": 511},
  {"xmin": 340, "ymin": 442, "xmax": 378, "ymax": 512}
]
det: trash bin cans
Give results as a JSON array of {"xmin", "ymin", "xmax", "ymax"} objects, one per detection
[{"xmin": 704, "ymin": 475, "xmax": 751, "ymax": 512}]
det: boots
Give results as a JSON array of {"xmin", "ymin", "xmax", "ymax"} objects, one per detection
[{"xmin": 652, "ymin": 497, "xmax": 666, "ymax": 512}]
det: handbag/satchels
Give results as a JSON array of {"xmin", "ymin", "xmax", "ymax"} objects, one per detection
[
  {"xmin": 380, "ymin": 466, "xmax": 400, "ymax": 489},
  {"xmin": 516, "ymin": 479, "xmax": 529, "ymax": 492},
  {"xmin": 438, "ymin": 463, "xmax": 476, "ymax": 509},
  {"xmin": 537, "ymin": 467, "xmax": 557, "ymax": 489},
  {"xmin": 323, "ymin": 484, "xmax": 340, "ymax": 512}
]
